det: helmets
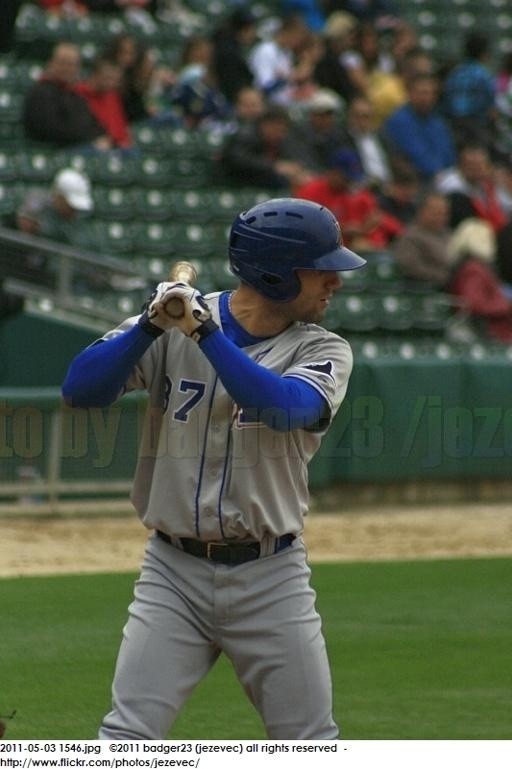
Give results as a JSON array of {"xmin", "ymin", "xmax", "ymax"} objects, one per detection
[{"xmin": 228, "ymin": 198, "xmax": 369, "ymax": 304}]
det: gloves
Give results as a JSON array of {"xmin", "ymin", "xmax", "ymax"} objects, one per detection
[{"xmin": 138, "ymin": 280, "xmax": 220, "ymax": 345}]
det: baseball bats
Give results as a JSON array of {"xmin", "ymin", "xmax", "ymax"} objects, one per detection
[{"xmin": 164, "ymin": 262, "xmax": 197, "ymax": 320}]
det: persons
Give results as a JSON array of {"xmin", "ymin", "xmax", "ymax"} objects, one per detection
[
  {"xmin": 1, "ymin": 0, "xmax": 512, "ymax": 342},
  {"xmin": 61, "ymin": 197, "xmax": 368, "ymax": 739}
]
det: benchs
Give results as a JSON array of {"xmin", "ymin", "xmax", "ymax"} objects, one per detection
[
  {"xmin": 331, "ymin": 249, "xmax": 508, "ymax": 360},
  {"xmin": 394, "ymin": 1, "xmax": 511, "ymax": 60},
  {"xmin": 0, "ymin": 1, "xmax": 273, "ymax": 324}
]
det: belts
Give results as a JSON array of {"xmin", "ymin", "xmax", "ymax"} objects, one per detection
[{"xmin": 155, "ymin": 530, "xmax": 296, "ymax": 567}]
